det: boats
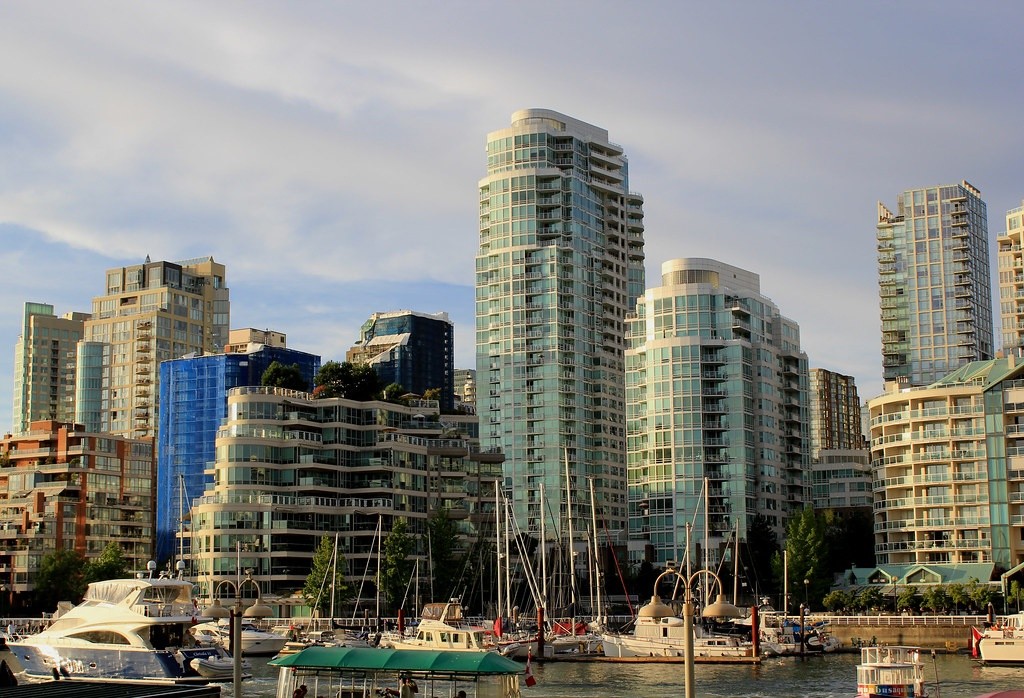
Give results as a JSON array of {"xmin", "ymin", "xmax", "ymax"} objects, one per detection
[
  {"xmin": 852, "ymin": 646, "xmax": 929, "ymax": 698},
  {"xmin": 967, "ymin": 610, "xmax": 1024, "ymax": 666}
]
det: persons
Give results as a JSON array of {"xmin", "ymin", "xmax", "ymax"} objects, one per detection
[
  {"xmin": 579, "ymin": 617, "xmax": 588, "ymax": 626},
  {"xmin": 292, "ymin": 633, "xmax": 312, "ymax": 644},
  {"xmin": 746, "ymin": 631, "xmax": 753, "ymax": 643},
  {"xmin": 453, "ymin": 691, "xmax": 466, "ymax": 698},
  {"xmin": 292, "ymin": 685, "xmax": 308, "ymax": 698},
  {"xmin": 787, "ymin": 594, "xmax": 794, "ymax": 616},
  {"xmin": 7, "ymin": 620, "xmax": 50, "ymax": 643},
  {"xmin": 182, "ymin": 629, "xmax": 204, "ymax": 650},
  {"xmin": 804, "ymin": 607, "xmax": 810, "ymax": 616},
  {"xmin": 398, "ymin": 671, "xmax": 419, "ymax": 698}
]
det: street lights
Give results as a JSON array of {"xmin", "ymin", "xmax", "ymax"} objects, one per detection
[
  {"xmin": 804, "ymin": 578, "xmax": 810, "ymax": 610},
  {"xmin": 891, "ymin": 576, "xmax": 898, "ymax": 616},
  {"xmin": 200, "ymin": 541, "xmax": 274, "ymax": 698}
]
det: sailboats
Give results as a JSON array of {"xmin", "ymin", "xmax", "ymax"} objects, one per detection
[{"xmin": 1, "ymin": 446, "xmax": 843, "ymax": 697}]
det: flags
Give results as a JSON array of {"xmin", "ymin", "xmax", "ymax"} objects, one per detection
[
  {"xmin": 524, "ymin": 652, "xmax": 536, "ymax": 687},
  {"xmin": 192, "ymin": 599, "xmax": 199, "ymax": 625},
  {"xmin": 971, "ymin": 627, "xmax": 984, "ymax": 658}
]
